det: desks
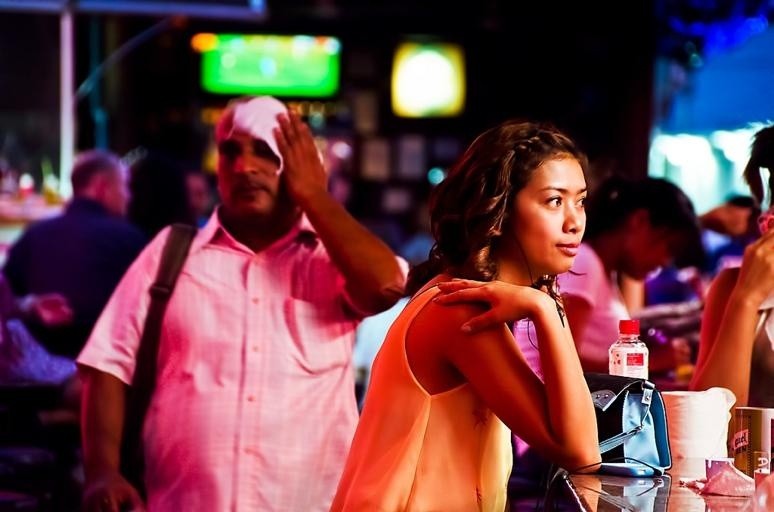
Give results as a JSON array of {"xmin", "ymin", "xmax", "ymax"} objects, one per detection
[{"xmin": 549, "ymin": 455, "xmax": 774, "ymax": 511}]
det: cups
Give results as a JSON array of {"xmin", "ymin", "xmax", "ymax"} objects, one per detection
[{"xmin": 728, "ymin": 407, "xmax": 772, "ymax": 482}]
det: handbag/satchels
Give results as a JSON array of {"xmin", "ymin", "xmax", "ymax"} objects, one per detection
[{"xmin": 583, "ymin": 373, "xmax": 672, "ymax": 471}]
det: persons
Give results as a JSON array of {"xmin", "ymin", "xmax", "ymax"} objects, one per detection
[
  {"xmin": 71, "ymin": 92, "xmax": 405, "ymax": 512},
  {"xmin": 328, "ymin": 119, "xmax": 603, "ymax": 512},
  {"xmin": 1, "ymin": 112, "xmax": 773, "ymax": 447}
]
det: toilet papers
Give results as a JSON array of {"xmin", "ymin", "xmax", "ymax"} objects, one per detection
[
  {"xmin": 661, "ymin": 385, "xmax": 737, "ymax": 475},
  {"xmin": 665, "ymin": 475, "xmax": 709, "ymax": 512}
]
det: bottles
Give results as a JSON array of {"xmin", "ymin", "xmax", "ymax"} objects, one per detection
[{"xmin": 607, "ymin": 319, "xmax": 648, "ymax": 382}]
